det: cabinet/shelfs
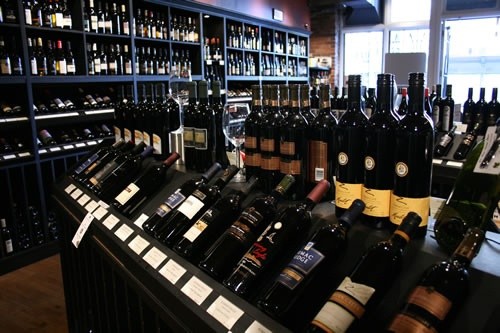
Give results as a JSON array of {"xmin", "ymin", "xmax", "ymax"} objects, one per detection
[{"xmin": 0, "ymin": 0, "xmax": 311, "ymax": 278}]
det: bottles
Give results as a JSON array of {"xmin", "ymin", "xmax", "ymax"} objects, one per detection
[{"xmin": 1, "ymin": 1, "xmax": 500, "ymax": 333}]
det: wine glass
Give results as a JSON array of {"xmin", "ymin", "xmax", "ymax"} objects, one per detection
[
  {"xmin": 222, "ymin": 103, "xmax": 246, "ymax": 183},
  {"xmin": 169, "ymin": 69, "xmax": 193, "ymax": 134}
]
